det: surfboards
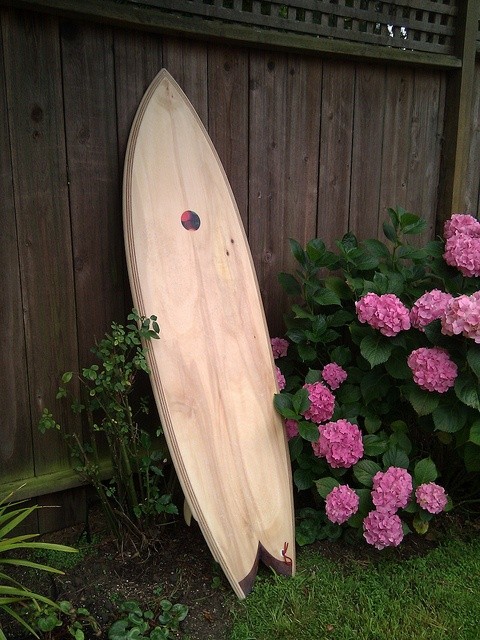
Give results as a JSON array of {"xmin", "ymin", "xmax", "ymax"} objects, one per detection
[{"xmin": 123, "ymin": 67, "xmax": 295, "ymax": 601}]
